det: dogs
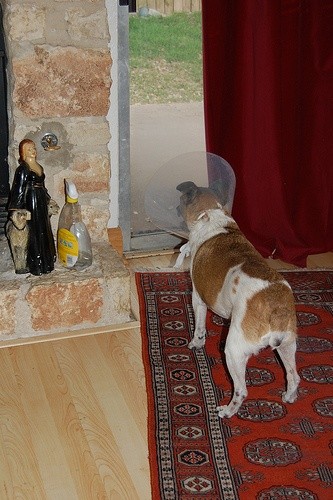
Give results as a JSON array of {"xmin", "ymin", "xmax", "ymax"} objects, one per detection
[{"xmin": 176, "ymin": 178, "xmax": 301, "ymax": 418}]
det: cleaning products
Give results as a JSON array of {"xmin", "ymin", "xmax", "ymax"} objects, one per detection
[{"xmin": 56, "ymin": 178, "xmax": 93, "ymax": 272}]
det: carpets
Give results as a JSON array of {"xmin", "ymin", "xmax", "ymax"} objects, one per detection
[{"xmin": 134, "ymin": 271, "xmax": 333, "ymax": 500}]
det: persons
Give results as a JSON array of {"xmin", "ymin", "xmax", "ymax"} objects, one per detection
[{"xmin": 5, "ymin": 138, "xmax": 58, "ymax": 276}]
